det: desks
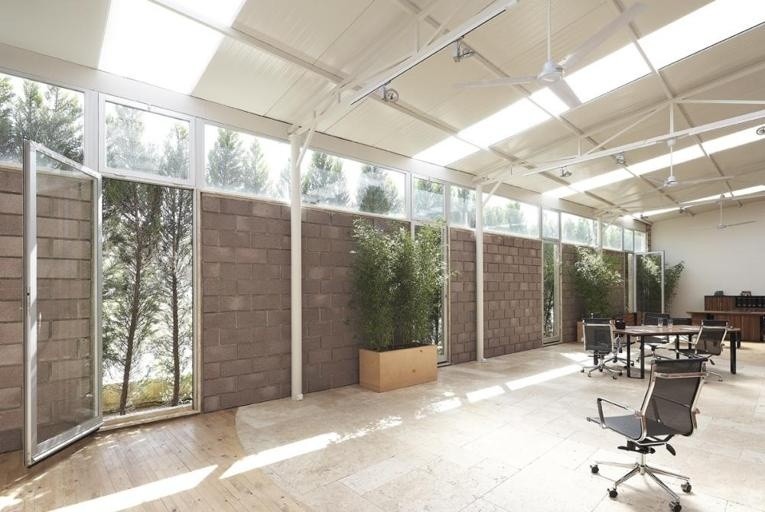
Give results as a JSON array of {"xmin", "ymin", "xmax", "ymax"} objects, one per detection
[
  {"xmin": 613, "ymin": 324, "xmax": 741, "ymax": 379},
  {"xmin": 686, "ymin": 309, "xmax": 765, "ymax": 343}
]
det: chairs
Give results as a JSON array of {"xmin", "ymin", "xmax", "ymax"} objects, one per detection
[
  {"xmin": 591, "ymin": 311, "xmax": 634, "ymax": 368},
  {"xmin": 637, "ymin": 312, "xmax": 670, "ymax": 363},
  {"xmin": 580, "ymin": 318, "xmax": 627, "ymax": 378},
  {"xmin": 669, "ymin": 320, "xmax": 728, "ymax": 383},
  {"xmin": 585, "ymin": 358, "xmax": 709, "ymax": 512}
]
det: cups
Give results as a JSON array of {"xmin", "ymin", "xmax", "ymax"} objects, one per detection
[
  {"xmin": 657, "ymin": 317, "xmax": 664, "ymax": 328},
  {"xmin": 667, "ymin": 319, "xmax": 674, "ymax": 329}
]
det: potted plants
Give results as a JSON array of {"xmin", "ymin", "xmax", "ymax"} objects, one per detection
[
  {"xmin": 574, "ymin": 245, "xmax": 624, "ymax": 343},
  {"xmin": 640, "ymin": 254, "xmax": 688, "ymax": 324},
  {"xmin": 349, "ymin": 215, "xmax": 461, "ymax": 393}
]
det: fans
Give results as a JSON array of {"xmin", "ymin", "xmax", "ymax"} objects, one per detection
[
  {"xmin": 618, "ymin": 138, "xmax": 734, "ymax": 200},
  {"xmin": 690, "ymin": 193, "xmax": 758, "ymax": 233},
  {"xmin": 456, "ymin": 0, "xmax": 652, "ymax": 109}
]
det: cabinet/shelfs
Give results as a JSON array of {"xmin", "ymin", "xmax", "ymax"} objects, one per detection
[{"xmin": 704, "ymin": 295, "xmax": 765, "ymax": 310}]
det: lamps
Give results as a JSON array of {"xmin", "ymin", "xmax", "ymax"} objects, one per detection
[
  {"xmin": 452, "ymin": 39, "xmax": 476, "ymax": 63},
  {"xmin": 559, "ymin": 167, "xmax": 572, "ymax": 178},
  {"xmin": 615, "ymin": 153, "xmax": 626, "ymax": 165},
  {"xmin": 378, "ymin": 85, "xmax": 398, "ymax": 104}
]
